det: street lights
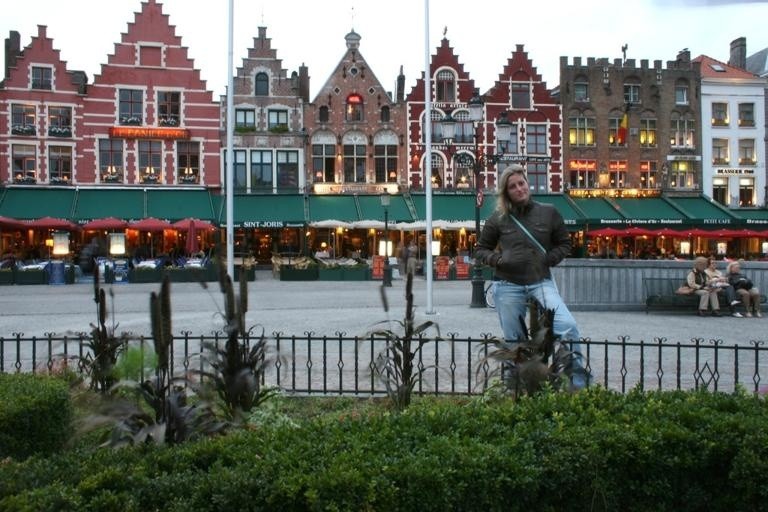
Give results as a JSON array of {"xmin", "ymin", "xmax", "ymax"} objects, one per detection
[
  {"xmin": 378, "ymin": 186, "xmax": 392, "ymax": 259},
  {"xmin": 438, "ymin": 87, "xmax": 514, "ymax": 309}
]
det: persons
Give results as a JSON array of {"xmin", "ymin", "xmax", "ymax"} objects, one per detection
[
  {"xmin": 468, "ymin": 165, "xmax": 587, "ymax": 388},
  {"xmin": 680, "ymin": 258, "xmax": 723, "ymax": 315},
  {"xmin": 725, "ymin": 260, "xmax": 764, "ymax": 320},
  {"xmin": 395, "ymin": 240, "xmax": 406, "ymax": 274},
  {"xmin": 704, "ymin": 256, "xmax": 744, "ymax": 320},
  {"xmin": 407, "ymin": 240, "xmax": 419, "ymax": 274}
]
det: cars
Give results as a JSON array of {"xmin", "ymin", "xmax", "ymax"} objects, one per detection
[{"xmin": 0, "ymin": 254, "xmax": 206, "ymax": 282}]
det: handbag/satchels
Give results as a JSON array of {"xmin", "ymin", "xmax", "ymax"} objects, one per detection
[
  {"xmin": 730, "ymin": 274, "xmax": 753, "ymax": 290},
  {"xmin": 714, "ymin": 277, "xmax": 730, "ymax": 287},
  {"xmin": 675, "ymin": 287, "xmax": 696, "ymax": 295}
]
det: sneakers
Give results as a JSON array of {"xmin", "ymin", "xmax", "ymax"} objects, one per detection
[
  {"xmin": 732, "ymin": 311, "xmax": 762, "ymax": 317},
  {"xmin": 731, "ymin": 300, "xmax": 741, "ymax": 306},
  {"xmin": 712, "ymin": 310, "xmax": 722, "ymax": 316},
  {"xmin": 698, "ymin": 311, "xmax": 704, "ymax": 316}
]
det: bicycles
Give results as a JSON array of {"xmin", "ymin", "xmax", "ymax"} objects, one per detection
[{"xmin": 483, "ymin": 279, "xmax": 496, "ymax": 308}]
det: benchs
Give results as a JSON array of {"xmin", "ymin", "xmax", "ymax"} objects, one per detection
[{"xmin": 642, "ymin": 278, "xmax": 766, "ymax": 313}]
